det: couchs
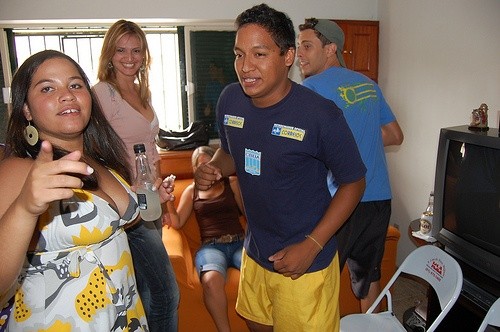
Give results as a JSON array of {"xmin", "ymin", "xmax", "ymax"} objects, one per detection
[{"xmin": 159, "ymin": 179, "xmax": 401, "ymax": 332}]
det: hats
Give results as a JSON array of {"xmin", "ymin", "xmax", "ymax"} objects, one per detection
[{"xmin": 307, "ymin": 19, "xmax": 347, "ymax": 67}]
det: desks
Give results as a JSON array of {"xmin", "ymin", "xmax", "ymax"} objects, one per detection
[{"xmin": 407, "ymin": 218, "xmax": 500, "ymax": 332}]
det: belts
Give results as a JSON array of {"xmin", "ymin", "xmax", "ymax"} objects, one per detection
[{"xmin": 203, "ymin": 235, "xmax": 244, "ymax": 243}]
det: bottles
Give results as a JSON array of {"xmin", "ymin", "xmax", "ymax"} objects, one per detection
[
  {"xmin": 426, "ymin": 194, "xmax": 435, "ymax": 212},
  {"xmin": 133, "ymin": 144, "xmax": 162, "ymax": 221}
]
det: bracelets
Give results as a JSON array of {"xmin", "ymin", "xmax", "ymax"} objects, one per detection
[{"xmin": 305, "ymin": 235, "xmax": 323, "ymax": 250}]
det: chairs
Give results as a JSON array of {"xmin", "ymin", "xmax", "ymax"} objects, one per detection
[{"xmin": 339, "ymin": 244, "xmax": 463, "ymax": 332}]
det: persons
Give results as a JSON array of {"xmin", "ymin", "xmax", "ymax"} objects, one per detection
[
  {"xmin": 163, "ymin": 145, "xmax": 245, "ymax": 332},
  {"xmin": 193, "ymin": 3, "xmax": 367, "ymax": 332},
  {"xmin": 0, "ymin": 50, "xmax": 176, "ymax": 332},
  {"xmin": 89, "ymin": 19, "xmax": 180, "ymax": 331},
  {"xmin": 295, "ymin": 18, "xmax": 403, "ymax": 314}
]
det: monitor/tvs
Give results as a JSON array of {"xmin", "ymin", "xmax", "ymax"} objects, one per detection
[{"xmin": 431, "ymin": 125, "xmax": 500, "ymax": 283}]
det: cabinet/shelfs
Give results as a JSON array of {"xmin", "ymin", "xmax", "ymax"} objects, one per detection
[{"xmin": 310, "ymin": 18, "xmax": 379, "ymax": 85}]
